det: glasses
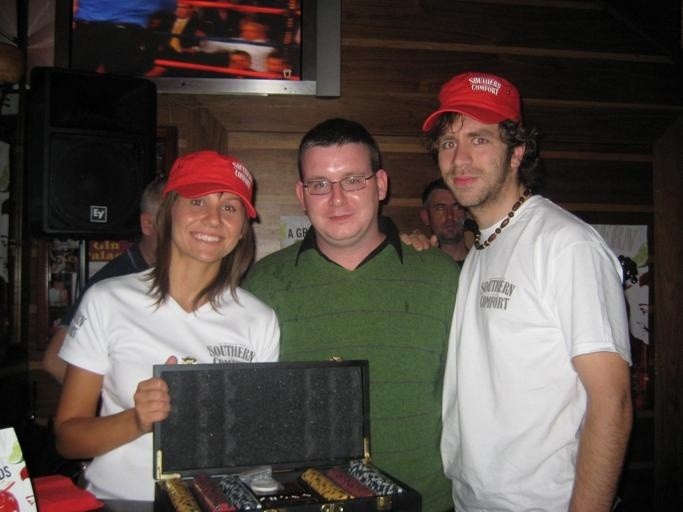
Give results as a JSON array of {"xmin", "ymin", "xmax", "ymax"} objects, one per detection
[{"xmin": 303, "ymin": 174, "xmax": 376, "ymax": 195}]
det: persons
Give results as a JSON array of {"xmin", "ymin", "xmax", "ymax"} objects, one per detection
[
  {"xmin": 238, "ymin": 119, "xmax": 463, "ymax": 511},
  {"xmin": 55, "ymin": 148, "xmax": 282, "ymax": 501},
  {"xmin": 395, "ymin": 71, "xmax": 635, "ymax": 512},
  {"xmin": 44, "ymin": 179, "xmax": 168, "ymax": 385},
  {"xmin": 72, "ymin": 0, "xmax": 299, "ymax": 80},
  {"xmin": 419, "ymin": 175, "xmax": 474, "ymax": 269}
]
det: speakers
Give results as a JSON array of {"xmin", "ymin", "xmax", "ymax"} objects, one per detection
[{"xmin": 29, "ymin": 65, "xmax": 157, "ymax": 240}]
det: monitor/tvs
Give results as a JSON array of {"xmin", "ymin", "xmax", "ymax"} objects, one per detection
[{"xmin": 55, "ymin": 0, "xmax": 317, "ymax": 96}]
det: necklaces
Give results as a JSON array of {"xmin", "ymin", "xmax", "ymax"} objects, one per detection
[{"xmin": 473, "ymin": 186, "xmax": 531, "ymax": 249}]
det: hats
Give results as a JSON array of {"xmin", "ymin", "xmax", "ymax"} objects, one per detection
[
  {"xmin": 422, "ymin": 71, "xmax": 521, "ymax": 132},
  {"xmin": 162, "ymin": 150, "xmax": 257, "ymax": 220}
]
